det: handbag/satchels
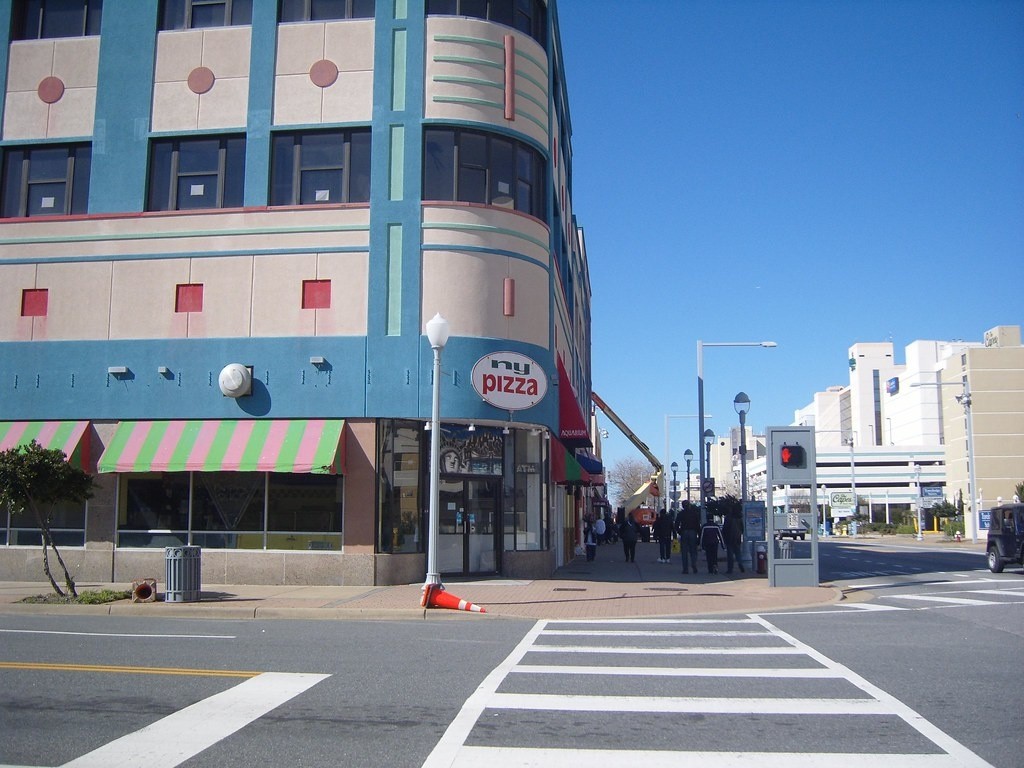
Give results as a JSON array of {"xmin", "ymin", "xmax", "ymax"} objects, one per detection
[{"xmin": 672, "ymin": 538, "xmax": 681, "ymax": 553}]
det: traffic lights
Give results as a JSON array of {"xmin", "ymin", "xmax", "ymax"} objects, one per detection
[{"xmin": 780, "ymin": 444, "xmax": 804, "ymax": 467}]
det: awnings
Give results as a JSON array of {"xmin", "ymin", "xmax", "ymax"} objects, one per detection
[
  {"xmin": 0, "ymin": 419, "xmax": 91, "ymax": 475},
  {"xmin": 96, "ymin": 419, "xmax": 348, "ymax": 476},
  {"xmin": 551, "ymin": 434, "xmax": 605, "ymax": 487}
]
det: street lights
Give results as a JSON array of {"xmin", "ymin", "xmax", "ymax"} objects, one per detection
[
  {"xmin": 697, "ymin": 340, "xmax": 777, "ymax": 524},
  {"xmin": 914, "ymin": 464, "xmax": 923, "ymax": 541},
  {"xmin": 734, "ymin": 392, "xmax": 754, "ymax": 566},
  {"xmin": 393, "ymin": 460, "xmax": 413, "ymax": 479},
  {"xmin": 420, "ymin": 313, "xmax": 450, "ymax": 607},
  {"xmin": 664, "ymin": 414, "xmax": 712, "ymax": 514},
  {"xmin": 704, "ymin": 429, "xmax": 715, "ymax": 501},
  {"xmin": 822, "ymin": 485, "xmax": 827, "ymax": 538},
  {"xmin": 684, "ymin": 449, "xmax": 694, "ymax": 501},
  {"xmin": 670, "ymin": 462, "xmax": 678, "ymax": 540},
  {"xmin": 910, "ymin": 381, "xmax": 978, "ymax": 545}
]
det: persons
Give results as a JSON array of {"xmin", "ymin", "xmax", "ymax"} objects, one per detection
[
  {"xmin": 699, "ymin": 513, "xmax": 725, "ymax": 574},
  {"xmin": 722, "ymin": 506, "xmax": 745, "ymax": 574},
  {"xmin": 595, "ymin": 516, "xmax": 619, "ymax": 545},
  {"xmin": 583, "ymin": 522, "xmax": 597, "ymax": 562},
  {"xmin": 652, "ymin": 509, "xmax": 677, "ymax": 563},
  {"xmin": 618, "ymin": 513, "xmax": 647, "ymax": 562},
  {"xmin": 675, "ymin": 500, "xmax": 701, "ymax": 576}
]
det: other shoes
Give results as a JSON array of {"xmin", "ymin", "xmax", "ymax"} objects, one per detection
[
  {"xmin": 656, "ymin": 558, "xmax": 665, "ymax": 563},
  {"xmin": 667, "ymin": 559, "xmax": 671, "ymax": 564},
  {"xmin": 738, "ymin": 563, "xmax": 745, "ymax": 573},
  {"xmin": 726, "ymin": 567, "xmax": 733, "ymax": 573},
  {"xmin": 691, "ymin": 564, "xmax": 697, "ymax": 573}
]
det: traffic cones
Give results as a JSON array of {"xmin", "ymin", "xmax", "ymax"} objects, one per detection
[
  {"xmin": 132, "ymin": 578, "xmax": 157, "ymax": 602},
  {"xmin": 422, "ymin": 583, "xmax": 486, "ymax": 613}
]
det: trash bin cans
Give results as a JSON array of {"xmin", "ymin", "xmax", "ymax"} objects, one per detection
[
  {"xmin": 165, "ymin": 546, "xmax": 201, "ymax": 603},
  {"xmin": 826, "ymin": 517, "xmax": 834, "ymax": 535},
  {"xmin": 586, "ymin": 543, "xmax": 597, "ymax": 560}
]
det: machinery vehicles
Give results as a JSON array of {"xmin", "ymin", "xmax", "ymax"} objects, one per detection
[{"xmin": 591, "ymin": 391, "xmax": 664, "ymax": 540}]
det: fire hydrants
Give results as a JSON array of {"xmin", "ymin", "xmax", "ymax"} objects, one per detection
[
  {"xmin": 756, "ymin": 546, "xmax": 768, "ymax": 574},
  {"xmin": 954, "ymin": 531, "xmax": 961, "ymax": 542}
]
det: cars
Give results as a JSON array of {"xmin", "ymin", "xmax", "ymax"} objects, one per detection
[
  {"xmin": 774, "ymin": 529, "xmax": 806, "ymax": 541},
  {"xmin": 985, "ymin": 504, "xmax": 1024, "ymax": 573}
]
